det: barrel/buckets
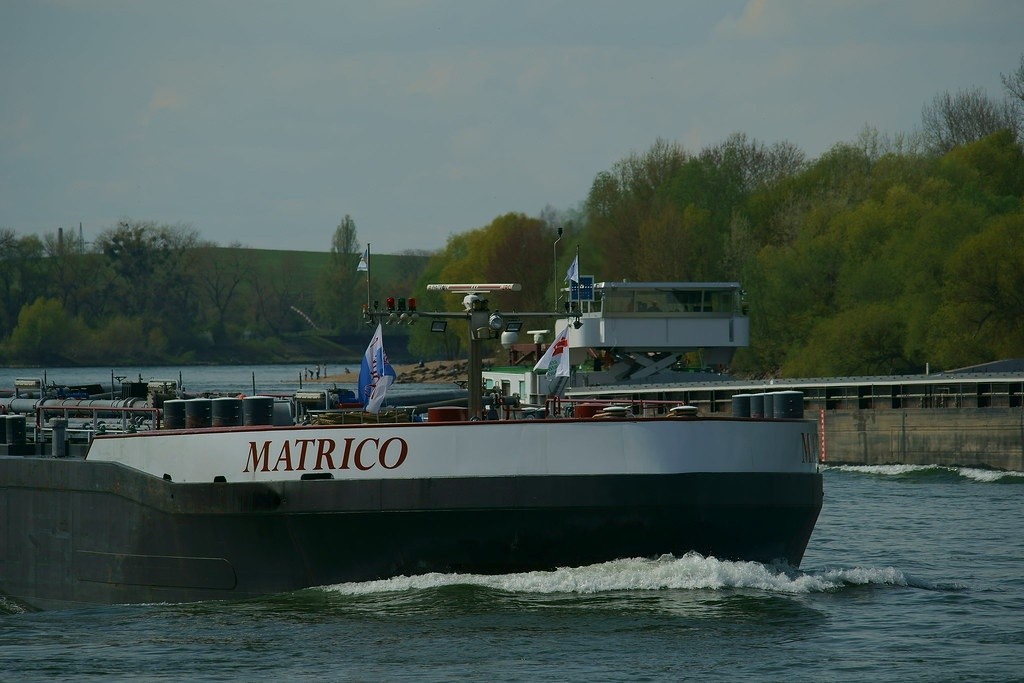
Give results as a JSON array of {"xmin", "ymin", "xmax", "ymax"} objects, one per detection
[
  {"xmin": 731, "ymin": 390, "xmax": 805, "ymax": 418},
  {"xmin": 574, "ymin": 402, "xmax": 610, "ymax": 418},
  {"xmin": 428, "ymin": 406, "xmax": 467, "ymax": 422},
  {"xmin": 0, "ymin": 415, "xmax": 27, "ymax": 445},
  {"xmin": 164, "ymin": 396, "xmax": 275, "ymax": 428}
]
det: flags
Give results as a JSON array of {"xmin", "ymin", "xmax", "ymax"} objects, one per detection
[
  {"xmin": 532, "ymin": 328, "xmax": 571, "ymax": 377},
  {"xmin": 359, "ymin": 323, "xmax": 395, "ymax": 414},
  {"xmin": 565, "ymin": 254, "xmax": 580, "ymax": 284},
  {"xmin": 357, "ymin": 249, "xmax": 369, "ymax": 273}
]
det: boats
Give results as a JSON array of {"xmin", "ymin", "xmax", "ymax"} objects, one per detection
[{"xmin": 0, "ymin": 228, "xmax": 825, "ymax": 606}]
